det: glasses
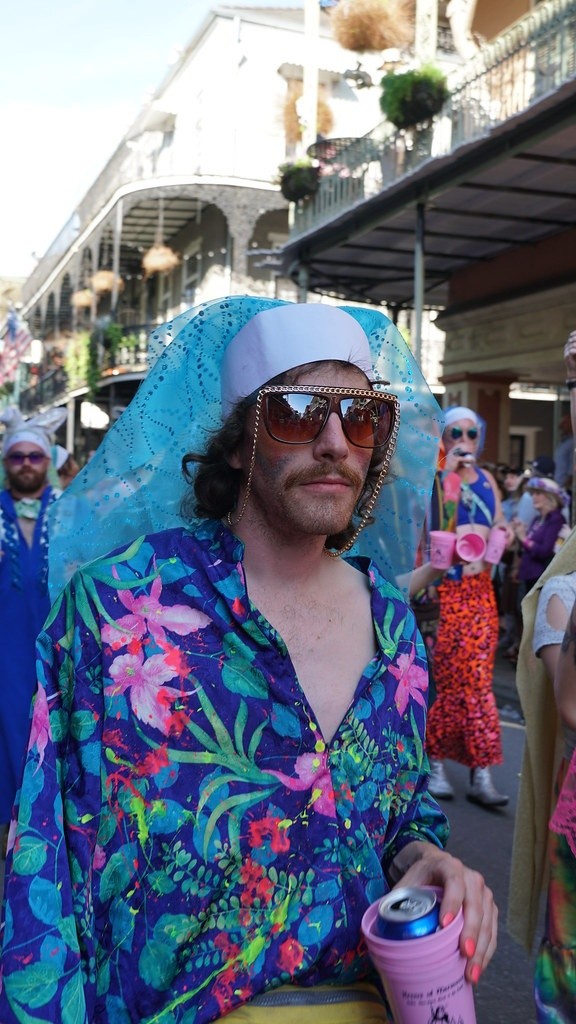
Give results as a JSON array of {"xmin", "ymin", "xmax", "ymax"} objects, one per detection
[
  {"xmin": 227, "ymin": 385, "xmax": 401, "ymax": 557},
  {"xmin": 2, "ymin": 451, "xmax": 48, "ymax": 465},
  {"xmin": 444, "ymin": 426, "xmax": 478, "ymax": 439}
]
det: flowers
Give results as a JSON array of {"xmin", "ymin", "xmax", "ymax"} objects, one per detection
[{"xmin": 24, "ymin": 561, "xmax": 432, "ymax": 1019}]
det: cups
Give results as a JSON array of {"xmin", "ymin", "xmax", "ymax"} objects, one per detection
[
  {"xmin": 485, "ymin": 529, "xmax": 508, "ymax": 565},
  {"xmin": 362, "ymin": 884, "xmax": 478, "ymax": 1024},
  {"xmin": 455, "ymin": 532, "xmax": 487, "ymax": 563},
  {"xmin": 429, "ymin": 529, "xmax": 457, "ymax": 569}
]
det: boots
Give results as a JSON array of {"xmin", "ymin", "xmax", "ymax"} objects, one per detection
[
  {"xmin": 465, "ymin": 767, "xmax": 509, "ymax": 806},
  {"xmin": 427, "ymin": 759, "xmax": 453, "ymax": 800}
]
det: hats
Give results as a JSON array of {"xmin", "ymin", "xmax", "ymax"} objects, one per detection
[
  {"xmin": 525, "ymin": 477, "xmax": 569, "ymax": 507},
  {"xmin": 441, "ymin": 405, "xmax": 486, "ymax": 455},
  {"xmin": 525, "ymin": 456, "xmax": 554, "ymax": 476},
  {"xmin": 0, "ymin": 405, "xmax": 68, "ymax": 488},
  {"xmin": 499, "ymin": 465, "xmax": 523, "ymax": 475},
  {"xmin": 46, "ymin": 295, "xmax": 446, "ymax": 607}
]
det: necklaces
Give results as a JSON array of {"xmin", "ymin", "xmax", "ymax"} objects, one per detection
[{"xmin": 9, "ymin": 495, "xmax": 45, "ymax": 520}]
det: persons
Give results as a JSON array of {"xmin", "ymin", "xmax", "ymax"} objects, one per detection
[
  {"xmin": 504, "ymin": 524, "xmax": 576, "ymax": 958},
  {"xmin": 50, "ymin": 443, "xmax": 78, "ymax": 489},
  {"xmin": 0, "ymin": 403, "xmax": 60, "ymax": 861},
  {"xmin": 533, "ymin": 595, "xmax": 576, "ymax": 1024},
  {"xmin": 0, "ymin": 296, "xmax": 501, "ymax": 1024},
  {"xmin": 481, "ymin": 454, "xmax": 572, "ymax": 665},
  {"xmin": 360, "ymin": 401, "xmax": 486, "ymax": 601},
  {"xmin": 413, "ymin": 405, "xmax": 510, "ymax": 808}
]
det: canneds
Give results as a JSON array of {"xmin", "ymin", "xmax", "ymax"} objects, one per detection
[{"xmin": 375, "ymin": 884, "xmax": 442, "ymax": 942}]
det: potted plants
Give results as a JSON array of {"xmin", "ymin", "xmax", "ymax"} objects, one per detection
[
  {"xmin": 381, "ymin": 64, "xmax": 446, "ymax": 128},
  {"xmin": 274, "ymin": 162, "xmax": 320, "ymax": 200}
]
